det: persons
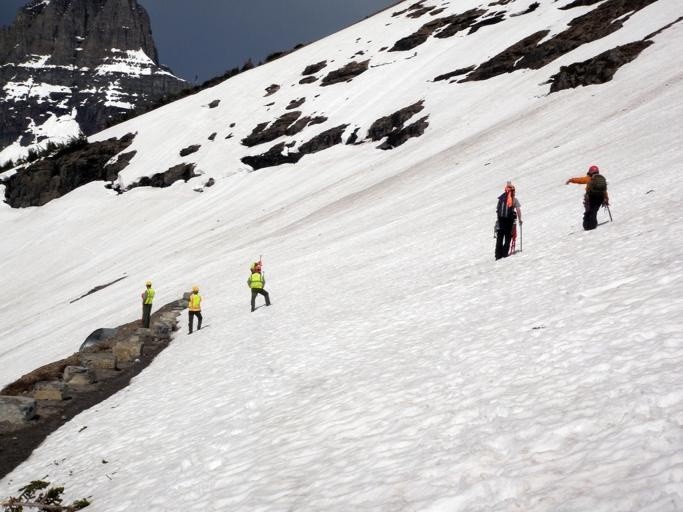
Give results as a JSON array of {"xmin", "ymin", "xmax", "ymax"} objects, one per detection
[
  {"xmin": 138, "ymin": 280, "xmax": 154, "ymax": 328},
  {"xmin": 493, "ymin": 185, "xmax": 522, "ymax": 260},
  {"xmin": 247, "ymin": 262, "xmax": 269, "ymax": 312},
  {"xmin": 185, "ymin": 285, "xmax": 202, "ymax": 334},
  {"xmin": 566, "ymin": 165, "xmax": 608, "ymax": 230}
]
